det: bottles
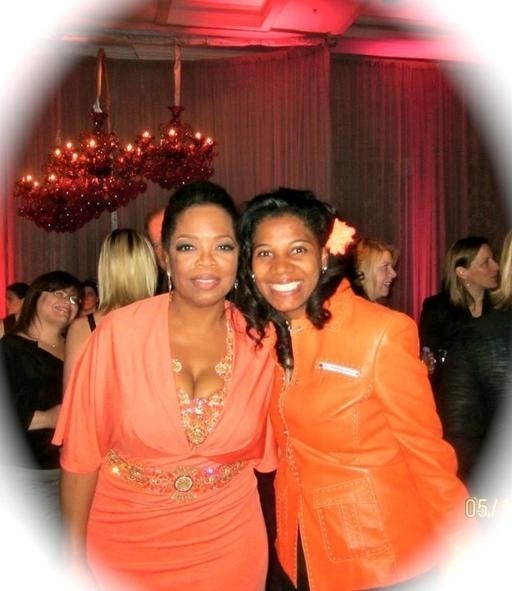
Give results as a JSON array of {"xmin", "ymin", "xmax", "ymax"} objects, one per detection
[{"xmin": 422, "ymin": 347, "xmax": 433, "ymax": 375}]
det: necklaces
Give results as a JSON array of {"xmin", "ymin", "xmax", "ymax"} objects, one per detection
[{"xmin": 22, "ymin": 329, "xmax": 63, "ymax": 348}]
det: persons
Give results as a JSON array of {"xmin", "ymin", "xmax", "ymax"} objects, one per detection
[
  {"xmin": 77, "ymin": 280, "xmax": 99, "ymax": 315},
  {"xmin": 63, "ymin": 227, "xmax": 157, "ymax": 394},
  {"xmin": 354, "ymin": 236, "xmax": 401, "ymax": 301},
  {"xmin": 420, "ymin": 230, "xmax": 511, "ymax": 482},
  {"xmin": 1, "ymin": 283, "xmax": 30, "ymax": 335},
  {"xmin": 233, "ymin": 187, "xmax": 475, "ymax": 591},
  {"xmin": 51, "ymin": 180, "xmax": 274, "ymax": 590},
  {"xmin": 1, "ymin": 270, "xmax": 85, "ymax": 552},
  {"xmin": 146, "ymin": 207, "xmax": 175, "ymax": 295}
]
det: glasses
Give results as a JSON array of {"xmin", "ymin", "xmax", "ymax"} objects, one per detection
[{"xmin": 40, "ymin": 289, "xmax": 79, "ymax": 305}]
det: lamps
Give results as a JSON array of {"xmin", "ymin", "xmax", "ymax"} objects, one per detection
[
  {"xmin": 13, "ymin": 112, "xmax": 143, "ymax": 238},
  {"xmin": 135, "ymin": 106, "xmax": 219, "ymax": 193}
]
